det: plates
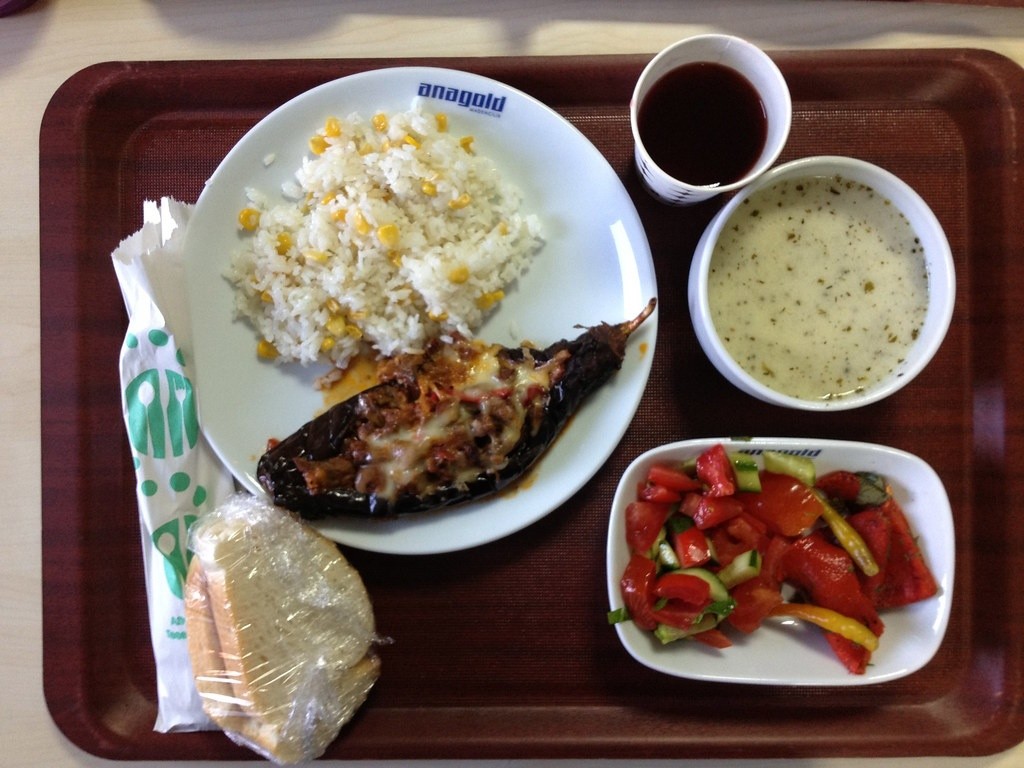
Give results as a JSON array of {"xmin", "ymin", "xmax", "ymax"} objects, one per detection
[
  {"xmin": 178, "ymin": 65, "xmax": 658, "ymax": 555},
  {"xmin": 606, "ymin": 433, "xmax": 959, "ymax": 687}
]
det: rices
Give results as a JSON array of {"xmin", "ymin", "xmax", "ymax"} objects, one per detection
[{"xmin": 214, "ymin": 95, "xmax": 544, "ymax": 390}]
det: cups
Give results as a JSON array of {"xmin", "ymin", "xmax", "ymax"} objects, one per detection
[{"xmin": 630, "ymin": 32, "xmax": 792, "ymax": 203}]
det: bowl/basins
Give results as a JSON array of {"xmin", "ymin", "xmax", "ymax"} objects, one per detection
[{"xmin": 686, "ymin": 154, "xmax": 958, "ymax": 413}]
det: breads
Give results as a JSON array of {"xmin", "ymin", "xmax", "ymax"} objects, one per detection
[{"xmin": 185, "ymin": 501, "xmax": 379, "ymax": 768}]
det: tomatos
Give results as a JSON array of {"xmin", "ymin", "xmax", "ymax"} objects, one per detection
[{"xmin": 621, "ymin": 444, "xmax": 934, "ymax": 674}]
